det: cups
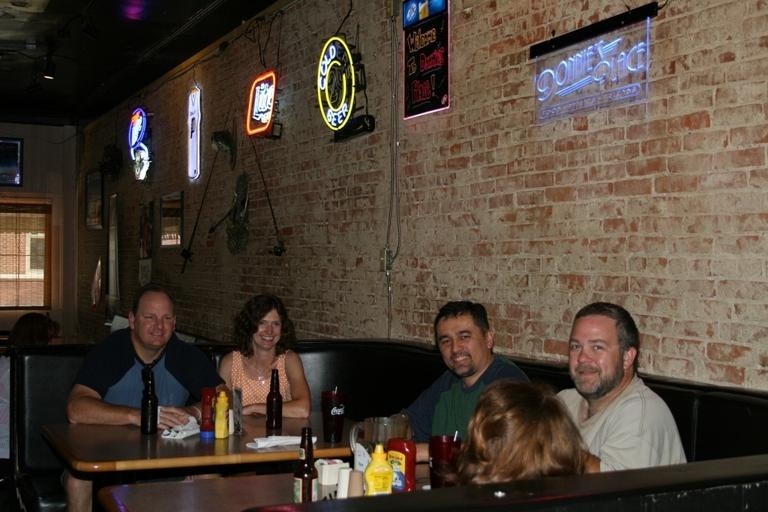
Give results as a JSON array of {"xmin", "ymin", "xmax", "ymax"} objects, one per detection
[
  {"xmin": 350, "ymin": 416, "xmax": 412, "ymax": 454},
  {"xmin": 429, "ymin": 435, "xmax": 462, "ymax": 490},
  {"xmin": 321, "ymin": 391, "xmax": 347, "ymax": 443}
]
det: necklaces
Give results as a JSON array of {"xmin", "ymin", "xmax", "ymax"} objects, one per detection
[{"xmin": 245, "ymin": 353, "xmax": 277, "ymax": 381}]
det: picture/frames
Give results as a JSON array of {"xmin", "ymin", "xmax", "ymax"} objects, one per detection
[
  {"xmin": 0, "ymin": 137, "xmax": 24, "ymax": 187},
  {"xmin": 85, "ymin": 169, "xmax": 105, "ymax": 230}
]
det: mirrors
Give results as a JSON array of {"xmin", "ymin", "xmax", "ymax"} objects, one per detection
[{"xmin": 159, "ymin": 191, "xmax": 184, "ymax": 249}]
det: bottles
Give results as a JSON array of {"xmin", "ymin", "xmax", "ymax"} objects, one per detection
[
  {"xmin": 199, "ymin": 383, "xmax": 242, "ymax": 439},
  {"xmin": 293, "ymin": 427, "xmax": 417, "ymax": 504},
  {"xmin": 266, "ymin": 369, "xmax": 283, "ymax": 436},
  {"xmin": 141, "ymin": 375, "xmax": 158, "ymax": 434}
]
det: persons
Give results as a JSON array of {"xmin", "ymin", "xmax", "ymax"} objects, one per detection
[
  {"xmin": 555, "ymin": 301, "xmax": 688, "ymax": 474},
  {"xmin": 63, "ymin": 283, "xmax": 234, "ymax": 511},
  {"xmin": 384, "ymin": 299, "xmax": 535, "ymax": 464},
  {"xmin": 434, "ymin": 378, "xmax": 591, "ymax": 485},
  {"xmin": 217, "ymin": 291, "xmax": 312, "ymax": 419},
  {"xmin": 7, "ymin": 312, "xmax": 60, "ymax": 348}
]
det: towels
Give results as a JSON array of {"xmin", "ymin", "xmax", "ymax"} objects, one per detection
[{"xmin": 246, "ymin": 435, "xmax": 317, "ymax": 450}]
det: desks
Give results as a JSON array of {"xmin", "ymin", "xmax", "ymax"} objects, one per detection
[{"xmin": 37, "ymin": 418, "xmax": 434, "ymax": 483}]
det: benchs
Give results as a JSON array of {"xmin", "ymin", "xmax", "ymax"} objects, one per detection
[
  {"xmin": 386, "ymin": 340, "xmax": 767, "ymax": 463},
  {"xmin": 254, "ymin": 454, "xmax": 767, "ymax": 510},
  {"xmin": 10, "ymin": 339, "xmax": 385, "ymax": 511}
]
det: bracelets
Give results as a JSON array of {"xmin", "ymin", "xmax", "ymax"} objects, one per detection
[{"xmin": 190, "ymin": 404, "xmax": 202, "ymax": 425}]
